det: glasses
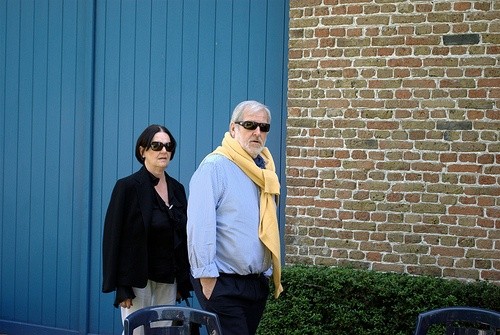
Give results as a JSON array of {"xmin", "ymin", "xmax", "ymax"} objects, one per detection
[
  {"xmin": 235, "ymin": 121, "xmax": 270, "ymax": 132},
  {"xmin": 151, "ymin": 142, "xmax": 175, "ymax": 152}
]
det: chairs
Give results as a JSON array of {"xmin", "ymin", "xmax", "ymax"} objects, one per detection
[
  {"xmin": 414, "ymin": 306, "xmax": 500, "ymax": 335},
  {"xmin": 124, "ymin": 304, "xmax": 223, "ymax": 335}
]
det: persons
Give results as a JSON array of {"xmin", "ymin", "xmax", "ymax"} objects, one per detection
[
  {"xmin": 102, "ymin": 124, "xmax": 194, "ymax": 335},
  {"xmin": 186, "ymin": 99, "xmax": 284, "ymax": 335}
]
